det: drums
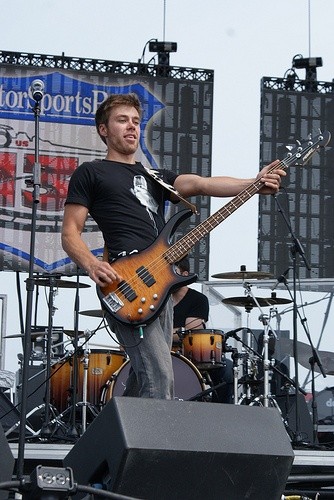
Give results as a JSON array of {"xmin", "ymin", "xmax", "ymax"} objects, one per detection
[
  {"xmin": 72, "ymin": 348, "xmax": 125, "ymax": 403},
  {"xmin": 51, "ymin": 360, "xmax": 71, "ymax": 409},
  {"xmin": 179, "ymin": 329, "xmax": 225, "ymax": 364}
]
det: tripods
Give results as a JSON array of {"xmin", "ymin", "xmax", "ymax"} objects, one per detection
[{"xmin": 3, "ymin": 286, "xmax": 109, "ymax": 443}]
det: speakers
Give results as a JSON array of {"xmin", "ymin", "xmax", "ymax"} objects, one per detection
[{"xmin": 63, "ymin": 396, "xmax": 295, "ymax": 500}]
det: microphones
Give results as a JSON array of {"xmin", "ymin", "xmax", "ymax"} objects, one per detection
[
  {"xmin": 242, "ymin": 281, "xmax": 252, "ymax": 287},
  {"xmin": 31, "ymin": 79, "xmax": 44, "ymax": 100},
  {"xmin": 270, "ymin": 267, "xmax": 290, "ymax": 290},
  {"xmin": 225, "ymin": 327, "xmax": 243, "ymax": 338}
]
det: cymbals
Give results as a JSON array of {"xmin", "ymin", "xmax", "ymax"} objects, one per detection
[
  {"xmin": 34, "ymin": 279, "xmax": 91, "ymax": 288},
  {"xmin": 78, "ymin": 309, "xmax": 104, "ymax": 318},
  {"xmin": 211, "ymin": 271, "xmax": 275, "ymax": 280},
  {"xmin": 266, "ymin": 297, "xmax": 292, "ymax": 305},
  {"xmin": 221, "ymin": 295, "xmax": 269, "ymax": 307}
]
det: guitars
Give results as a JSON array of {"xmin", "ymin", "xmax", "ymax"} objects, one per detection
[{"xmin": 94, "ymin": 128, "xmax": 327, "ymax": 328}]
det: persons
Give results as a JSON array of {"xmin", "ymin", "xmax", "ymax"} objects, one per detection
[
  {"xmin": 61, "ymin": 92, "xmax": 287, "ymax": 400},
  {"xmin": 171, "ymin": 256, "xmax": 209, "ymax": 341}
]
